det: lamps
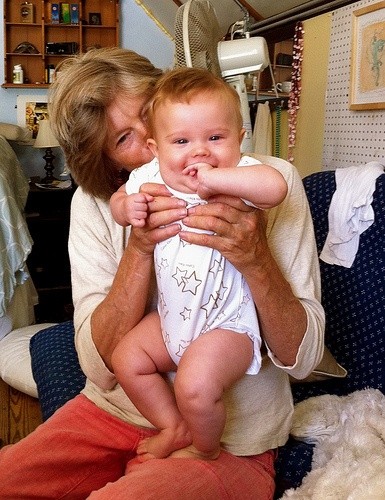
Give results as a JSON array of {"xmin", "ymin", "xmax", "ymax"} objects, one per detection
[{"xmin": 33, "ymin": 120, "xmax": 61, "ymax": 184}]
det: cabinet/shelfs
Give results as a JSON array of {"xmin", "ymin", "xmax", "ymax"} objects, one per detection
[
  {"xmin": 26, "ymin": 180, "xmax": 76, "ymax": 281},
  {"xmin": 250, "ymin": 39, "xmax": 292, "ymax": 97},
  {"xmin": 1, "ymin": 0, "xmax": 120, "ymax": 89}
]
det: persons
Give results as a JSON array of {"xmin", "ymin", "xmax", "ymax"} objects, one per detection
[
  {"xmin": 110, "ymin": 68, "xmax": 289, "ymax": 467},
  {"xmin": 0, "ymin": 46, "xmax": 324, "ymax": 500}
]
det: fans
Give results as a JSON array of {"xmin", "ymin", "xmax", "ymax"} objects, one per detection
[{"xmin": 173, "ymin": 0, "xmax": 271, "ymax": 155}]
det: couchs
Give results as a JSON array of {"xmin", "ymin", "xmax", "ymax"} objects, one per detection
[{"xmin": 29, "ymin": 169, "xmax": 385, "ymax": 500}]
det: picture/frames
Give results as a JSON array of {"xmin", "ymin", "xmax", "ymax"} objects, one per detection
[
  {"xmin": 349, "ymin": 1, "xmax": 385, "ymax": 111},
  {"xmin": 17, "ymin": 95, "xmax": 48, "ymax": 146},
  {"xmin": 88, "ymin": 12, "xmax": 101, "ymax": 25}
]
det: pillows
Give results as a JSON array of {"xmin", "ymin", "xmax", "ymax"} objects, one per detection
[{"xmin": 0, "ymin": 323, "xmax": 57, "ymax": 398}]
